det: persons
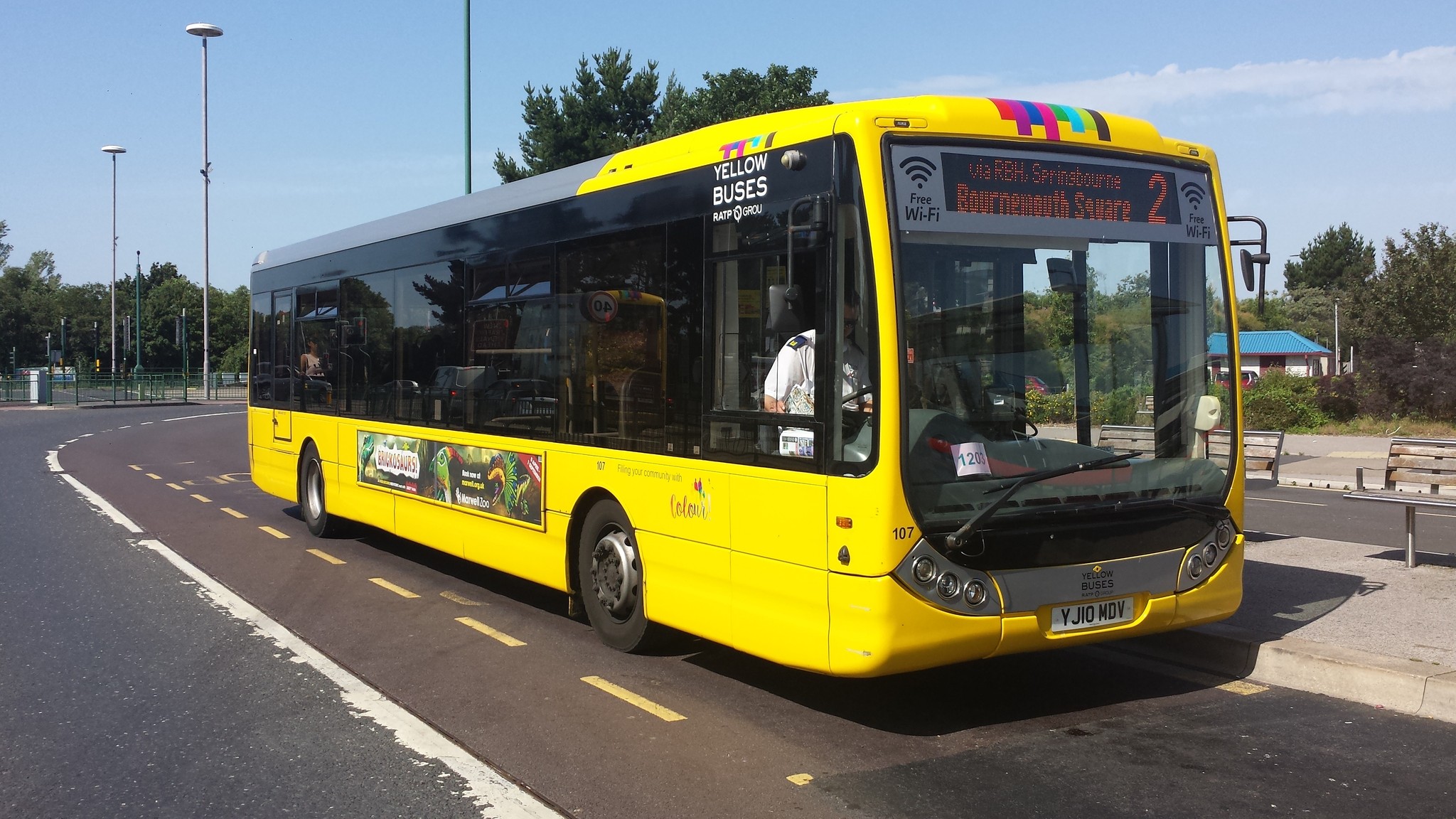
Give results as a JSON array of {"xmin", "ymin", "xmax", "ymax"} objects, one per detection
[
  {"xmin": 764, "ymin": 287, "xmax": 873, "ymax": 432},
  {"xmin": 301, "ymin": 335, "xmax": 326, "ymax": 381}
]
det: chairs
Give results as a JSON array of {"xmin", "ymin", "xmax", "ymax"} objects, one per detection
[{"xmin": 754, "ymin": 310, "xmax": 806, "ymax": 456}]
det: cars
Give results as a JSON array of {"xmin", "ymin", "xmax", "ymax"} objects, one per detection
[
  {"xmin": 1214, "ymin": 371, "xmax": 1231, "ymax": 390},
  {"xmin": 1241, "ymin": 370, "xmax": 1259, "ymax": 391},
  {"xmin": 370, "ymin": 381, "xmax": 418, "ymax": 399},
  {"xmin": 470, "ymin": 378, "xmax": 557, "ymax": 427},
  {"xmin": 257, "ymin": 361, "xmax": 333, "ymax": 402},
  {"xmin": 1025, "ymin": 375, "xmax": 1050, "ymax": 398}
]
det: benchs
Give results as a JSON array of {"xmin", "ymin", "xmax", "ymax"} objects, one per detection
[
  {"xmin": 1098, "ymin": 424, "xmax": 1284, "ymax": 490},
  {"xmin": 1136, "ymin": 396, "xmax": 1154, "ymax": 414},
  {"xmin": 1342, "ymin": 437, "xmax": 1456, "ymax": 567}
]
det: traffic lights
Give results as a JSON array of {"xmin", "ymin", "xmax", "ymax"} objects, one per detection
[{"xmin": 353, "ymin": 316, "xmax": 368, "ymax": 346}]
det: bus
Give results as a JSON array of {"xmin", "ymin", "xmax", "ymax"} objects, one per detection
[
  {"xmin": 471, "ymin": 290, "xmax": 667, "ymax": 433},
  {"xmin": 248, "ymin": 96, "xmax": 1269, "ymax": 683}
]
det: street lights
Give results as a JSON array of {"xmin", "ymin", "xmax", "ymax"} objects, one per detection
[
  {"xmin": 101, "ymin": 143, "xmax": 127, "ymax": 379},
  {"xmin": 186, "ymin": 22, "xmax": 224, "ymax": 401}
]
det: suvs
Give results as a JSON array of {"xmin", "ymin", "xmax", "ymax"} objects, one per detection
[{"xmin": 423, "ymin": 365, "xmax": 496, "ymax": 419}]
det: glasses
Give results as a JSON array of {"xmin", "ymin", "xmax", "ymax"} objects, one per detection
[{"xmin": 844, "ymin": 315, "xmax": 859, "ymax": 328}]
